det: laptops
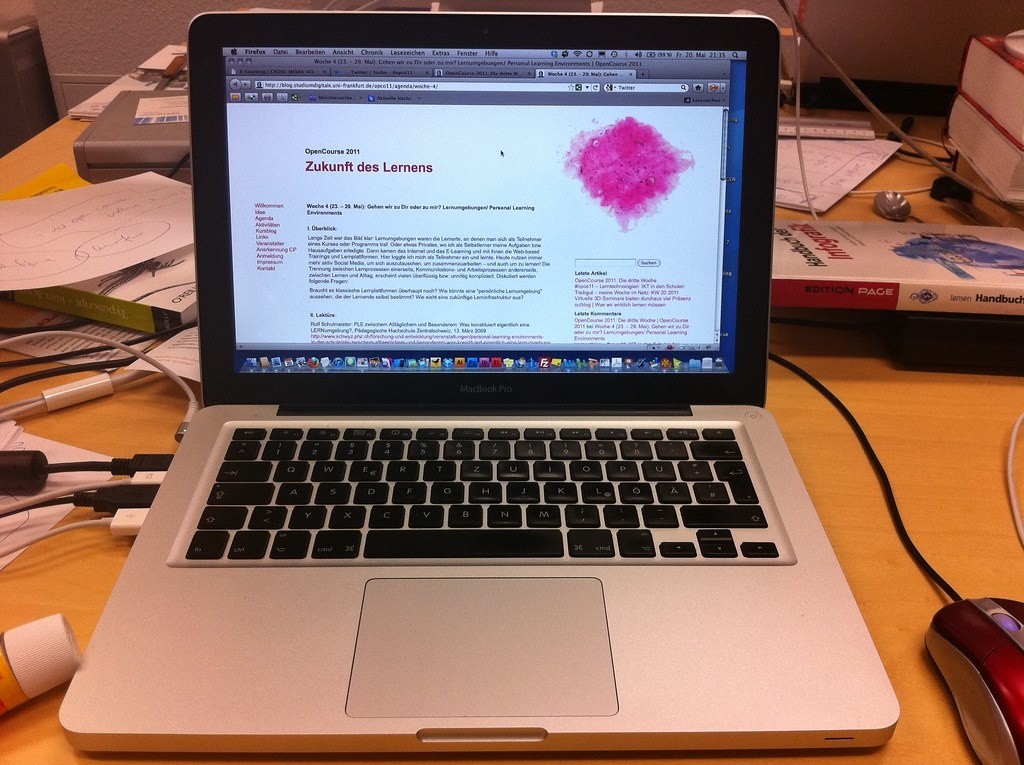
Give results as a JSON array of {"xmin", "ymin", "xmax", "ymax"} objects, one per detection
[{"xmin": 59, "ymin": 10, "xmax": 902, "ymax": 758}]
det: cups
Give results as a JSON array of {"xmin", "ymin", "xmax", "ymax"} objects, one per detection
[{"xmin": 0, "ymin": 613, "xmax": 83, "ymax": 717}]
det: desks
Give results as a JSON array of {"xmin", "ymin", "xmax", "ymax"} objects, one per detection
[{"xmin": 0, "ymin": 108, "xmax": 1024, "ymax": 765}]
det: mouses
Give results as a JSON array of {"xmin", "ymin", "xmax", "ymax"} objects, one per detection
[{"xmin": 926, "ymin": 594, "xmax": 1024, "ymax": 765}]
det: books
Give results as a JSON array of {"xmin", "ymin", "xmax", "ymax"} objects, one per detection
[
  {"xmin": 68, "ymin": 67, "xmax": 188, "ymax": 122},
  {"xmin": 948, "ymin": 33, "xmax": 1023, "ymax": 205},
  {"xmin": 764, "ymin": 218, "xmax": 1024, "ymax": 315},
  {"xmin": 0, "ymin": 185, "xmax": 208, "ymax": 336}
]
